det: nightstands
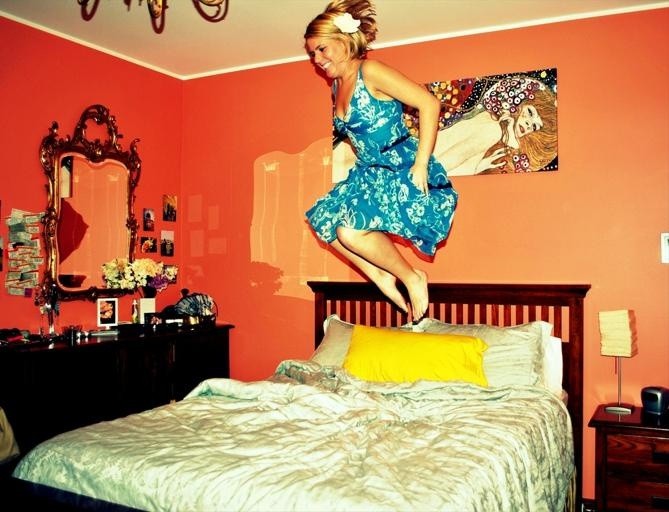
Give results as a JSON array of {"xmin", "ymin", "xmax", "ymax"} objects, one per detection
[{"xmin": 588, "ymin": 403, "xmax": 668, "ymax": 512}]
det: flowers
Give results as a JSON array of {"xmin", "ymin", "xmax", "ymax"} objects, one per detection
[{"xmin": 100, "ymin": 256, "xmax": 178, "ymax": 298}]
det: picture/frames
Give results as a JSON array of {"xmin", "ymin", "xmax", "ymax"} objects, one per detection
[{"xmin": 97, "ymin": 296, "xmax": 119, "ymax": 326}]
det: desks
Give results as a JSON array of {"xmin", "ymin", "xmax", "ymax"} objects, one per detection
[{"xmin": 0, "ymin": 322, "xmax": 237, "ymax": 445}]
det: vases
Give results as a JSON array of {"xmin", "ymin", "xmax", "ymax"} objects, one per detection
[{"xmin": 137, "ymin": 297, "xmax": 157, "ymax": 325}]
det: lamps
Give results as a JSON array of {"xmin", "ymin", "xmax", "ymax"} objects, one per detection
[{"xmin": 597, "ymin": 309, "xmax": 638, "ymax": 414}]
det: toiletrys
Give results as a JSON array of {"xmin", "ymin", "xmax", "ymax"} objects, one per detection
[{"xmin": 65, "ymin": 324, "xmax": 77, "ymax": 341}]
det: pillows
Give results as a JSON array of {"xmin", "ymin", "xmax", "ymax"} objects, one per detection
[
  {"xmin": 307, "ymin": 314, "xmax": 422, "ymax": 370},
  {"xmin": 417, "ymin": 315, "xmax": 554, "ymax": 391},
  {"xmin": 544, "ymin": 336, "xmax": 563, "ymax": 394},
  {"xmin": 342, "ymin": 322, "xmax": 490, "ymax": 389}
]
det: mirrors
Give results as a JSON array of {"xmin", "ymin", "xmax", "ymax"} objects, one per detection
[{"xmin": 36, "ymin": 99, "xmax": 143, "ymax": 303}]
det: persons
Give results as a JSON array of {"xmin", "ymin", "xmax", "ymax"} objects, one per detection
[
  {"xmin": 305, "ymin": 0, "xmax": 458, "ymax": 323},
  {"xmin": 405, "ymin": 85, "xmax": 559, "ymax": 175}
]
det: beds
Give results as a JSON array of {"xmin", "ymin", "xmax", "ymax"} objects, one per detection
[{"xmin": 6, "ymin": 280, "xmax": 593, "ymax": 511}]
